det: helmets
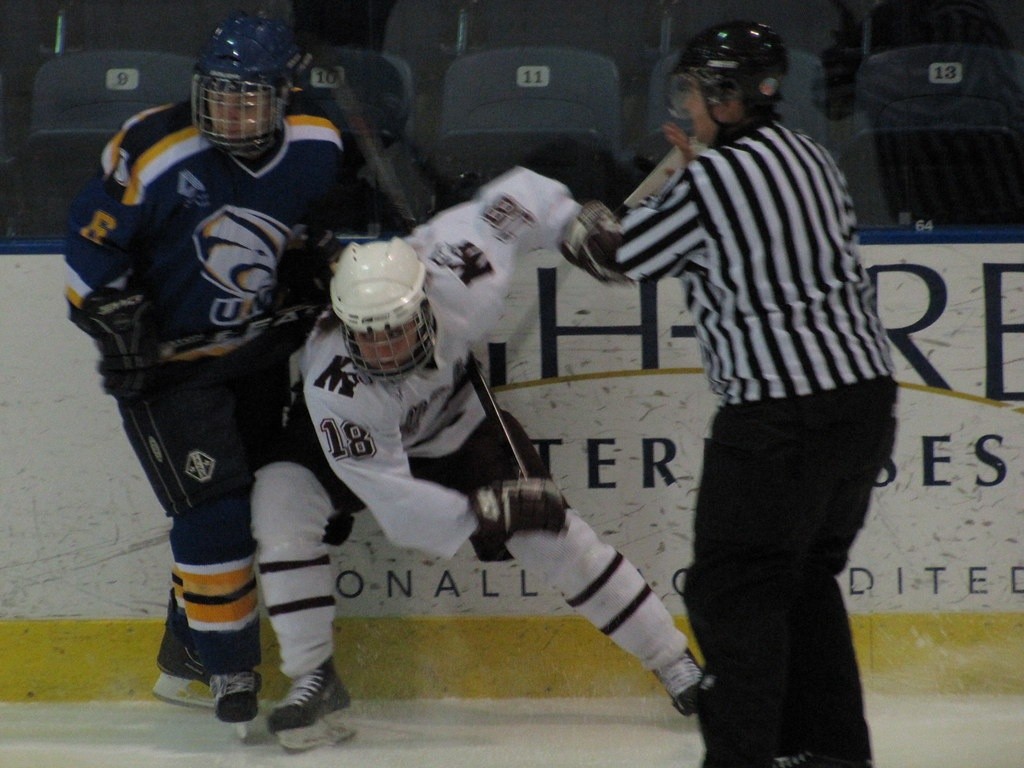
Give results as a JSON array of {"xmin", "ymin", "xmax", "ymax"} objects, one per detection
[
  {"xmin": 190, "ymin": 11, "xmax": 302, "ymax": 159},
  {"xmin": 331, "ymin": 237, "xmax": 434, "ymax": 382},
  {"xmin": 666, "ymin": 21, "xmax": 789, "ymax": 118}
]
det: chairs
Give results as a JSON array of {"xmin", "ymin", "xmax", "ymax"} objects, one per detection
[{"xmin": 16, "ymin": 1, "xmax": 1024, "ymax": 238}]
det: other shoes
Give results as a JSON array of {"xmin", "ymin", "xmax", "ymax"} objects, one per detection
[{"xmin": 772, "ymin": 753, "xmax": 871, "ymax": 768}]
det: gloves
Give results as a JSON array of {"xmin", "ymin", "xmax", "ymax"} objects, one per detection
[
  {"xmin": 79, "ymin": 277, "xmax": 158, "ymax": 402},
  {"xmin": 562, "ymin": 202, "xmax": 622, "ymax": 284},
  {"xmin": 471, "ymin": 478, "xmax": 565, "ymax": 556}
]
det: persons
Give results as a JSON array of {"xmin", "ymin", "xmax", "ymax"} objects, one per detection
[
  {"xmin": 813, "ymin": 0, "xmax": 1024, "ymax": 229},
  {"xmin": 559, "ymin": 20, "xmax": 898, "ymax": 768},
  {"xmin": 251, "ymin": 165, "xmax": 702, "ymax": 753},
  {"xmin": 64, "ymin": 9, "xmax": 345, "ymax": 742}
]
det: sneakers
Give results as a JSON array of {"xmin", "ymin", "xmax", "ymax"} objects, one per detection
[
  {"xmin": 206, "ymin": 667, "xmax": 261, "ymax": 739},
  {"xmin": 652, "ymin": 648, "xmax": 705, "ymax": 716},
  {"xmin": 151, "ymin": 588, "xmax": 215, "ymax": 709},
  {"xmin": 267, "ymin": 657, "xmax": 357, "ymax": 750}
]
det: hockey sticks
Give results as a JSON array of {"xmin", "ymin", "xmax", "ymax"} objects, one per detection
[
  {"xmin": 611, "ymin": 133, "xmax": 708, "ymax": 229},
  {"xmin": 326, "ymin": 70, "xmax": 534, "ymax": 483}
]
